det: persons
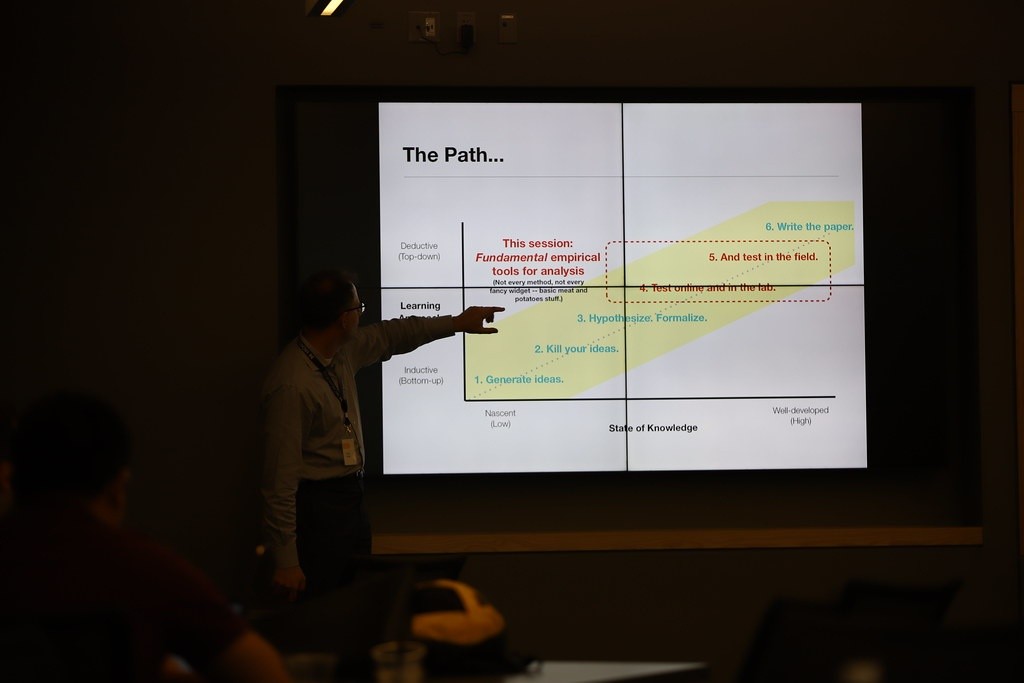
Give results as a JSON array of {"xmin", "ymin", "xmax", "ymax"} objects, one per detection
[
  {"xmin": 0, "ymin": 392, "xmax": 290, "ymax": 683},
  {"xmin": 265, "ymin": 270, "xmax": 505, "ymax": 683}
]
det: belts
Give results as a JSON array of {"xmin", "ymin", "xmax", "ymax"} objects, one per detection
[{"xmin": 343, "ymin": 469, "xmax": 367, "ymax": 483}]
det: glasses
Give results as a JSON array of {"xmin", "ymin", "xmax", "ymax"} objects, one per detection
[{"xmin": 336, "ymin": 303, "xmax": 366, "ymax": 318}]
